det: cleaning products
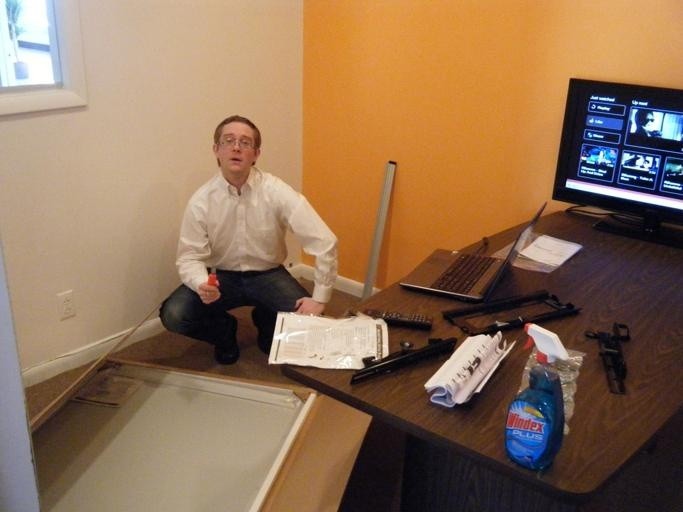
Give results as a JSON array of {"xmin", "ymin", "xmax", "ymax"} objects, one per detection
[{"xmin": 504, "ymin": 322, "xmax": 571, "ymax": 472}]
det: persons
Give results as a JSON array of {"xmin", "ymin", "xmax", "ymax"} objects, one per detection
[
  {"xmin": 157, "ymin": 113, "xmax": 341, "ymax": 366},
  {"xmin": 634, "ymin": 110, "xmax": 662, "ymax": 138}
]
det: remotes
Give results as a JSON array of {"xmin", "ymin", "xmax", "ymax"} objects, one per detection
[{"xmin": 363, "ymin": 308, "xmax": 433, "ymax": 330}]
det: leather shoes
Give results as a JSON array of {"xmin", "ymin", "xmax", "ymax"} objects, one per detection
[
  {"xmin": 252, "ymin": 305, "xmax": 276, "ymax": 354},
  {"xmin": 215, "ymin": 314, "xmax": 238, "ymax": 364}
]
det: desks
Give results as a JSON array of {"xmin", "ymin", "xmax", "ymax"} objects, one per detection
[{"xmin": 280, "ymin": 212, "xmax": 683, "ymax": 512}]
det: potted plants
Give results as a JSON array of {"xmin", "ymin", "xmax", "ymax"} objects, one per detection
[{"xmin": 5, "ymin": 0, "xmax": 27, "ymax": 80}]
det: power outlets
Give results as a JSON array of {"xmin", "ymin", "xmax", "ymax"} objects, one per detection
[{"xmin": 56, "ymin": 289, "xmax": 76, "ymax": 321}]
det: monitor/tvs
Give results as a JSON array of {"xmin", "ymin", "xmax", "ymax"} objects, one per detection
[{"xmin": 552, "ymin": 78, "xmax": 683, "ymax": 249}]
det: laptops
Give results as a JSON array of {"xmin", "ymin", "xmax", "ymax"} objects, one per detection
[{"xmin": 399, "ymin": 202, "xmax": 548, "ymax": 303}]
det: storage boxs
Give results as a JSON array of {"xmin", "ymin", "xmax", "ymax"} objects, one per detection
[{"xmin": 31, "ymin": 283, "xmax": 373, "ymax": 512}]
